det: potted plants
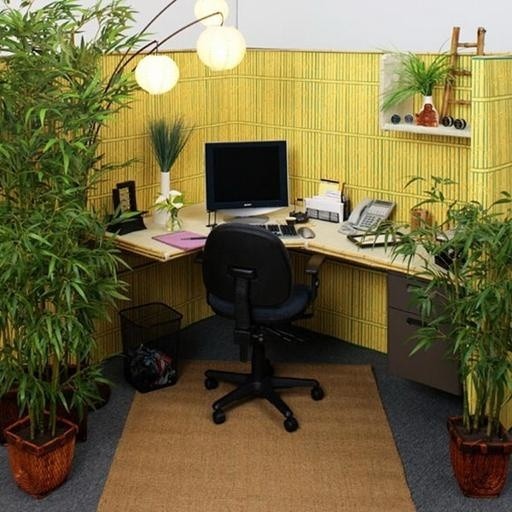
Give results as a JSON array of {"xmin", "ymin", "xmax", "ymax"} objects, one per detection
[
  {"xmin": 0, "ymin": 1, "xmax": 157, "ymax": 496},
  {"xmin": 141, "ymin": 118, "xmax": 200, "ymax": 227},
  {"xmin": 352, "ymin": 168, "xmax": 511, "ymax": 494},
  {"xmin": 380, "ymin": 43, "xmax": 473, "ymax": 125}
]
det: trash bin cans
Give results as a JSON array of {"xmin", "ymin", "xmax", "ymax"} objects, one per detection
[{"xmin": 118, "ymin": 302, "xmax": 183, "ymax": 393}]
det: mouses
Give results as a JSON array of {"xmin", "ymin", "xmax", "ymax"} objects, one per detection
[{"xmin": 298, "ymin": 227, "xmax": 315, "ymax": 238}]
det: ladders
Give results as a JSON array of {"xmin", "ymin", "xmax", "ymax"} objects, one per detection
[{"xmin": 439, "ymin": 27, "xmax": 486, "ymax": 124}]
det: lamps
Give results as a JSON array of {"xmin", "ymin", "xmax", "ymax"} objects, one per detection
[{"xmin": 63, "ymin": 0, "xmax": 246, "ymax": 413}]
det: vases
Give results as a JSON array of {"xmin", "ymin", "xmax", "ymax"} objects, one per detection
[{"xmin": 166, "ymin": 217, "xmax": 183, "ymax": 232}]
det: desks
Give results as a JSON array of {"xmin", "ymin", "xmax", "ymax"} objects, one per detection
[{"xmin": 85, "ymin": 207, "xmax": 468, "ymax": 397}]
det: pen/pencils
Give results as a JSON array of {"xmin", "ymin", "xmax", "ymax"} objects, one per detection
[{"xmin": 181, "ymin": 237, "xmax": 208, "ymax": 240}]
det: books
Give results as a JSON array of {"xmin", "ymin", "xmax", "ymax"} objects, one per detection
[{"xmin": 152, "ymin": 229, "xmax": 208, "ymax": 252}]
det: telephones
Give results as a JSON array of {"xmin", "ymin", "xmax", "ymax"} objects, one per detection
[{"xmin": 347, "ymin": 198, "xmax": 396, "ymax": 232}]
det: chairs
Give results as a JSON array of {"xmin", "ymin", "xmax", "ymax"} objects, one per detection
[{"xmin": 201, "ymin": 223, "xmax": 326, "ymax": 433}]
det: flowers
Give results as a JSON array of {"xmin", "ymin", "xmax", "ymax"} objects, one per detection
[{"xmin": 155, "ymin": 186, "xmax": 191, "ymax": 229}]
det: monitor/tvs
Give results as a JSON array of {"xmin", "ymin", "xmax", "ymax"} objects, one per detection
[{"xmin": 205, "ymin": 139, "xmax": 290, "ymax": 224}]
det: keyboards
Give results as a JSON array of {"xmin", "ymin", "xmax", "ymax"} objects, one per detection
[{"xmin": 251, "ymin": 224, "xmax": 298, "ymax": 237}]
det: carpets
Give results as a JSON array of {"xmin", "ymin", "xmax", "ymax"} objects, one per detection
[{"xmin": 98, "ymin": 363, "xmax": 416, "ymax": 510}]
